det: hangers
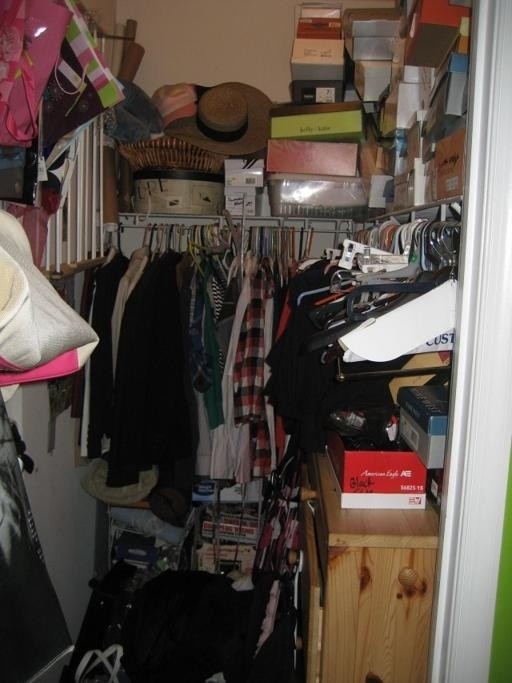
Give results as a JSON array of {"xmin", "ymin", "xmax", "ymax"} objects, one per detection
[
  {"xmin": 106, "ymin": 223, "xmax": 312, "ymax": 287},
  {"xmin": 297, "ymin": 217, "xmax": 458, "ymax": 385}
]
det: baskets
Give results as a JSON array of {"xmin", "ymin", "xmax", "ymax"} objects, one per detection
[{"xmin": 116, "ymin": 137, "xmax": 230, "ymax": 172}]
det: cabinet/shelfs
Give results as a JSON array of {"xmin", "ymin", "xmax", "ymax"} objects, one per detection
[
  {"xmin": 100, "ymin": 494, "xmax": 260, "ymax": 574},
  {"xmin": 287, "ymin": 447, "xmax": 441, "ymax": 683}
]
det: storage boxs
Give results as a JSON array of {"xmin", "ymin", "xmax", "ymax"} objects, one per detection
[
  {"xmin": 222, "ymin": 155, "xmax": 267, "ymax": 214},
  {"xmin": 266, "ymin": 2, "xmax": 473, "ymax": 215},
  {"xmin": 400, "ymin": 384, "xmax": 453, "ymax": 467},
  {"xmin": 328, "ymin": 413, "xmax": 424, "ymax": 509}
]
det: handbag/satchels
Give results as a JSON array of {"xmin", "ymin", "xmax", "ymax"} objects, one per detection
[
  {"xmin": 0, "ymin": 0, "xmax": 126, "ymax": 211},
  {"xmin": 79, "ymin": 457, "xmax": 159, "ymax": 506}
]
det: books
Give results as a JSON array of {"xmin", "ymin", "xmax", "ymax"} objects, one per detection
[
  {"xmin": 124, "ymin": 536, "xmax": 157, "ymax": 572},
  {"xmin": 200, "ymin": 502, "xmax": 259, "ymax": 578}
]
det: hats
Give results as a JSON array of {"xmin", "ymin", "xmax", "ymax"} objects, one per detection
[{"xmin": 112, "ymin": 82, "xmax": 273, "ymax": 156}]
